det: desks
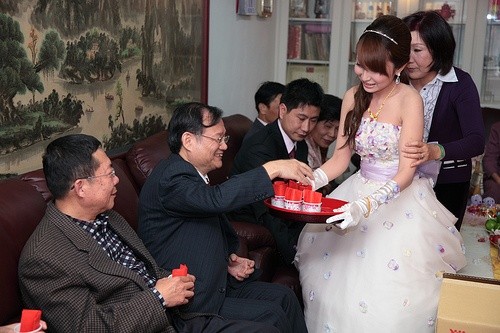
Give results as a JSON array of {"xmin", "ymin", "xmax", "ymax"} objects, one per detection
[{"xmin": 436, "ymin": 204, "xmax": 500, "ymax": 333}]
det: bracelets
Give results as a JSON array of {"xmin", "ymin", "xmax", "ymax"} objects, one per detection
[
  {"xmin": 365, "ymin": 198, "xmax": 370, "ymax": 218},
  {"xmin": 437, "ymin": 144, "xmax": 445, "ymax": 161}
]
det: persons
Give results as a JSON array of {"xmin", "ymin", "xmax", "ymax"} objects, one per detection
[
  {"xmin": 482, "ymin": 138, "xmax": 500, "ymax": 205},
  {"xmin": 402, "ymin": 10, "xmax": 486, "ymax": 232},
  {"xmin": 229, "ymin": 78, "xmax": 344, "ymax": 250},
  {"xmin": 294, "ymin": 15, "xmax": 468, "ymax": 333},
  {"xmin": 137, "ymin": 101, "xmax": 315, "ymax": 333},
  {"xmin": 17, "ymin": 133, "xmax": 280, "ymax": 333}
]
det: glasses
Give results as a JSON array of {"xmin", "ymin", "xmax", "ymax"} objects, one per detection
[
  {"xmin": 189, "ymin": 132, "xmax": 229, "ymax": 145},
  {"xmin": 71, "ymin": 168, "xmax": 115, "ymax": 190}
]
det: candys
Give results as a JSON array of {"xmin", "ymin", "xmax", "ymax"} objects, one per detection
[{"xmin": 468, "ymin": 203, "xmax": 500, "ymax": 236}]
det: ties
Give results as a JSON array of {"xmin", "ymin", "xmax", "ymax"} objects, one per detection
[{"xmin": 289, "ymin": 146, "xmax": 296, "ymax": 159}]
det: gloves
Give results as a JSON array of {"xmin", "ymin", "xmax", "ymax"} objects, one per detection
[
  {"xmin": 297, "ymin": 167, "xmax": 329, "ymax": 192},
  {"xmin": 325, "ymin": 180, "xmax": 400, "ymax": 230}
]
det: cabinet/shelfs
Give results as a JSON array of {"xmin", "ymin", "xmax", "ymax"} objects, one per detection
[{"xmin": 285, "ymin": 0, "xmax": 500, "ymax": 109}]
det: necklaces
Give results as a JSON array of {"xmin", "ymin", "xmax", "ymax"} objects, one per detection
[{"xmin": 367, "ymin": 85, "xmax": 396, "ymax": 121}]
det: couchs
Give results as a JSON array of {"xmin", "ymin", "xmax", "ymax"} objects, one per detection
[{"xmin": 0, "ymin": 112, "xmax": 299, "ymax": 333}]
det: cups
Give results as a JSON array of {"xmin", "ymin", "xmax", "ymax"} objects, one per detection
[
  {"xmin": 301, "ymin": 201, "xmax": 322, "ymax": 213},
  {"xmin": 283, "ymin": 199, "xmax": 303, "ymax": 211},
  {"xmin": 270, "ymin": 195, "xmax": 285, "ymax": 209}
]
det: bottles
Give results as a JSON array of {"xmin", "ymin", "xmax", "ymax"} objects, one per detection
[{"xmin": 13, "ymin": 322, "xmax": 42, "ymax": 333}]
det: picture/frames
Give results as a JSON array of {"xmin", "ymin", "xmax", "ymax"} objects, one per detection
[{"xmin": 0, "ymin": 0, "xmax": 210, "ymax": 180}]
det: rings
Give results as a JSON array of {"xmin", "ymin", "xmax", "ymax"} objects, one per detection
[{"xmin": 420, "ymin": 154, "xmax": 423, "ymax": 159}]
